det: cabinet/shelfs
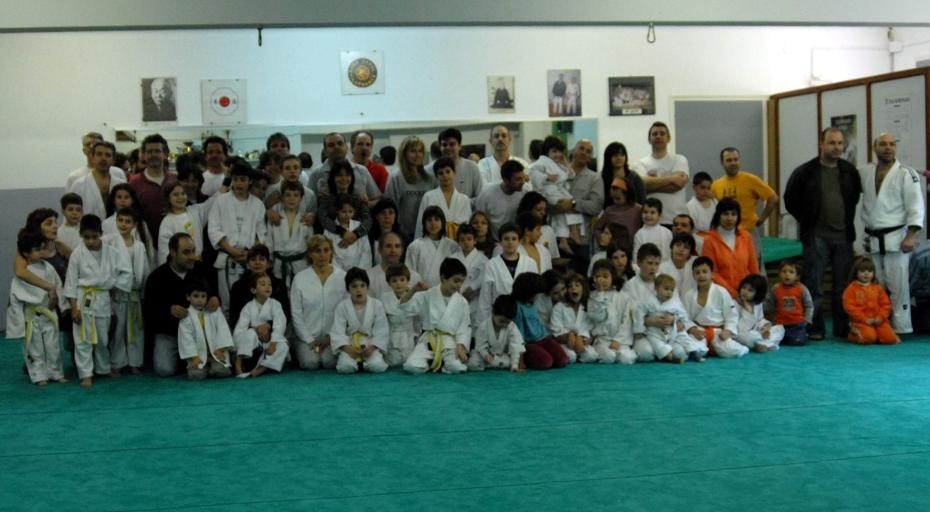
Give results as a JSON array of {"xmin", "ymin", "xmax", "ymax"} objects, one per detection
[{"xmin": 768, "ymin": 66, "xmax": 929, "ymax": 242}]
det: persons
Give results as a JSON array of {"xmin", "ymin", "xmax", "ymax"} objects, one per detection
[
  {"xmin": 856, "ymin": 131, "xmax": 926, "ymax": 335},
  {"xmin": 143, "ymin": 78, "xmax": 175, "ymax": 120},
  {"xmin": 783, "ymin": 126, "xmax": 862, "ymax": 342},
  {"xmin": 551, "ymin": 73, "xmax": 567, "ymax": 115},
  {"xmin": 489, "ymin": 80, "xmax": 515, "ymax": 108},
  {"xmin": 2, "ymin": 120, "xmax": 815, "ymax": 390},
  {"xmin": 840, "ymin": 256, "xmax": 902, "ymax": 345},
  {"xmin": 565, "ymin": 76, "xmax": 580, "ymax": 115},
  {"xmin": 612, "ymin": 84, "xmax": 653, "ymax": 114}
]
